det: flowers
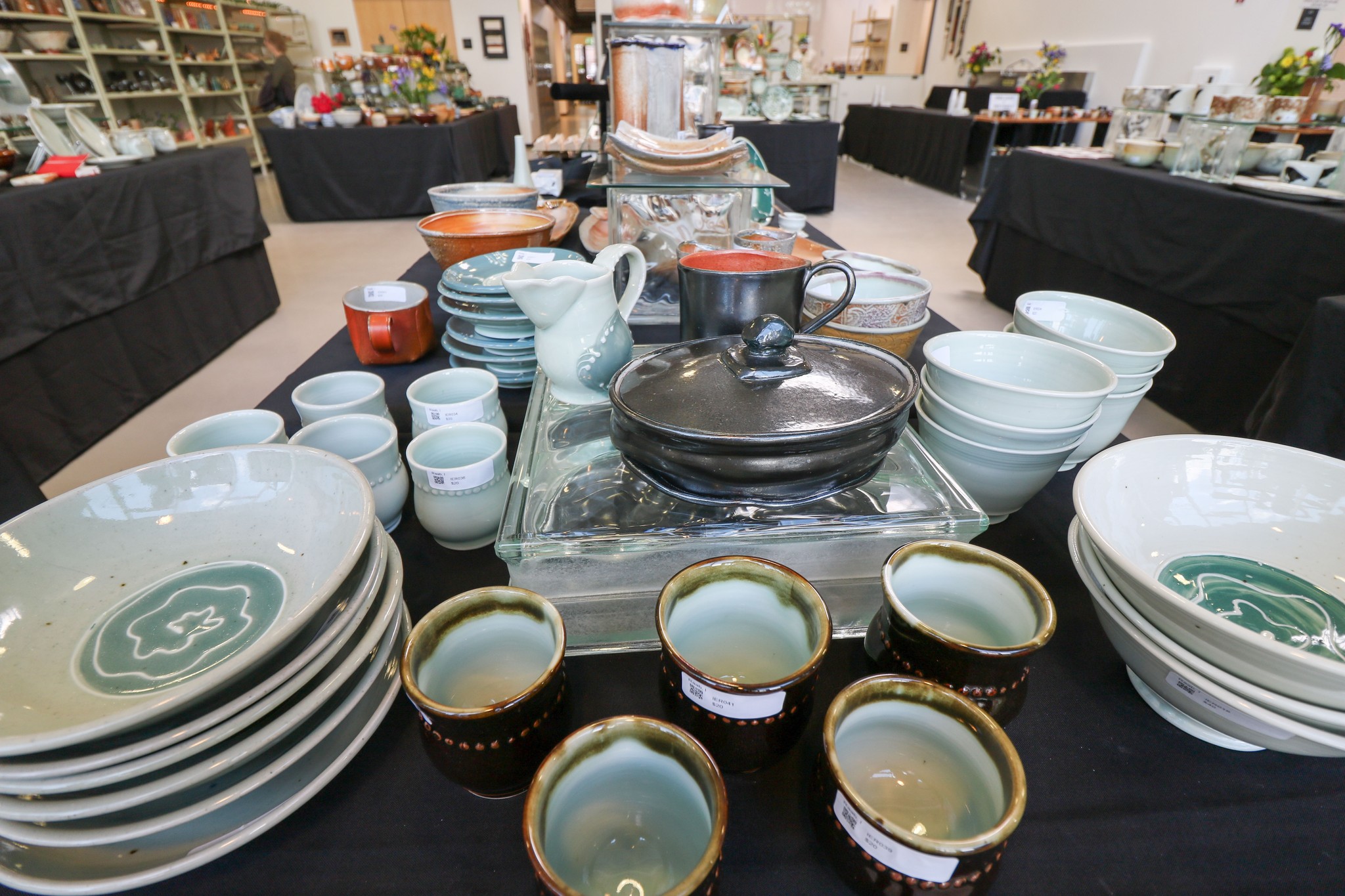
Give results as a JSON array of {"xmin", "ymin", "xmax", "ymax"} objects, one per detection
[
  {"xmin": 1016, "ymin": 40, "xmax": 1065, "ymax": 99},
  {"xmin": 958, "ymin": 42, "xmax": 1002, "ymax": 75},
  {"xmin": 382, "ymin": 24, "xmax": 451, "ymax": 102},
  {"xmin": 1249, "ymin": 22, "xmax": 1345, "ymax": 96}
]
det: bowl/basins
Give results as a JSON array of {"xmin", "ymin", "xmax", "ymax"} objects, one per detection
[
  {"xmin": 1257, "ymin": 141, "xmax": 1304, "ymax": 175},
  {"xmin": 268, "ymin": 106, "xmax": 295, "ymax": 127},
  {"xmin": 802, "ymin": 249, "xmax": 933, "ymax": 360},
  {"xmin": 1116, "ymin": 139, "xmax": 1164, "ymax": 167},
  {"xmin": 1067, "ymin": 434, "xmax": 1345, "ymax": 757},
  {"xmin": 1164, "ymin": 143, "xmax": 1210, "ymax": 171},
  {"xmin": 0, "ymin": 30, "xmax": 70, "ymax": 52},
  {"xmin": 725, "ymin": 79, "xmax": 746, "ymax": 93},
  {"xmin": 1238, "ymin": 141, "xmax": 1269, "ymax": 172},
  {"xmin": 416, "ymin": 180, "xmax": 555, "ymax": 271},
  {"xmin": 764, "ymin": 53, "xmax": 788, "ymax": 71},
  {"xmin": 915, "ymin": 290, "xmax": 1176, "ymax": 525},
  {"xmin": 301, "ymin": 111, "xmax": 361, "ymax": 129}
]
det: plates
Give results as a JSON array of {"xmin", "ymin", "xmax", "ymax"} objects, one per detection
[
  {"xmin": 294, "ymin": 83, "xmax": 314, "ymax": 126},
  {"xmin": 538, "ymin": 199, "xmax": 641, "ymax": 255},
  {"xmin": 27, "ymin": 107, "xmax": 146, "ymax": 169},
  {"xmin": 603, "ymin": 120, "xmax": 750, "ymax": 175},
  {"xmin": 734, "ymin": 37, "xmax": 758, "ymax": 70},
  {"xmin": 785, "ymin": 60, "xmax": 803, "ymax": 81},
  {"xmin": 732, "ymin": 136, "xmax": 775, "ymax": 226},
  {"xmin": 0, "ymin": 443, "xmax": 411, "ymax": 896},
  {"xmin": 438, "ymin": 247, "xmax": 587, "ymax": 389},
  {"xmin": 719, "ymin": 75, "xmax": 794, "ymax": 122}
]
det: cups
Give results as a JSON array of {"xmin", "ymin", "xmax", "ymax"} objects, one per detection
[
  {"xmin": 860, "ymin": 539, "xmax": 1057, "ymax": 731},
  {"xmin": 522, "ymin": 715, "xmax": 729, "ymax": 896},
  {"xmin": 822, "ymin": 673, "xmax": 1027, "ymax": 896},
  {"xmin": 655, "ymin": 555, "xmax": 833, "ymax": 774},
  {"xmin": 287, "ymin": 413, "xmax": 409, "ymax": 533},
  {"xmin": 1121, "ymin": 65, "xmax": 1345, "ymax": 187},
  {"xmin": 342, "ymin": 281, "xmax": 435, "ymax": 366},
  {"xmin": 291, "ymin": 371, "xmax": 395, "ymax": 428},
  {"xmin": 406, "ymin": 367, "xmax": 508, "ymax": 439},
  {"xmin": 406, "ymin": 422, "xmax": 511, "ymax": 551},
  {"xmin": 166, "ymin": 409, "xmax": 289, "ymax": 457},
  {"xmin": 677, "ymin": 229, "xmax": 857, "ymax": 342},
  {"xmin": 113, "ymin": 127, "xmax": 177, "ymax": 162},
  {"xmin": 778, "ymin": 212, "xmax": 809, "ymax": 238},
  {"xmin": 501, "ymin": 243, "xmax": 647, "ymax": 405},
  {"xmin": 400, "ymin": 585, "xmax": 566, "ymax": 799}
]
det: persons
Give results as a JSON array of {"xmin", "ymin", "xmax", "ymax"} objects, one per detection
[{"xmin": 255, "ymin": 30, "xmax": 296, "ymax": 112}]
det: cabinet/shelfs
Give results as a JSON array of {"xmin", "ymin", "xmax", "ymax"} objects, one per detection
[
  {"xmin": 782, "ymin": 84, "xmax": 831, "ymax": 119},
  {"xmin": 0, "ymin": 0, "xmax": 316, "ymax": 168},
  {"xmin": 845, "ymin": 6, "xmax": 894, "ymax": 75}
]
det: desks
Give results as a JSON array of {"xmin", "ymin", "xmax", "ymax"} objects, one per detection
[
  {"xmin": 837, "ymin": 104, "xmax": 1111, "ymax": 200},
  {"xmin": 0, "ymin": 186, "xmax": 1345, "ymax": 896},
  {"xmin": 258, "ymin": 106, "xmax": 520, "ymax": 224},
  {"xmin": 0, "ymin": 146, "xmax": 282, "ymax": 524},
  {"xmin": 967, "ymin": 146, "xmax": 1345, "ymax": 461},
  {"xmin": 725, "ymin": 120, "xmax": 840, "ymax": 214}
]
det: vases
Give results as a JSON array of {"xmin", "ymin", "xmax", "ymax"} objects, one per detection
[
  {"xmin": 968, "ymin": 74, "xmax": 977, "ymax": 87},
  {"xmin": 1299, "ymin": 76, "xmax": 1326, "ymax": 123}
]
